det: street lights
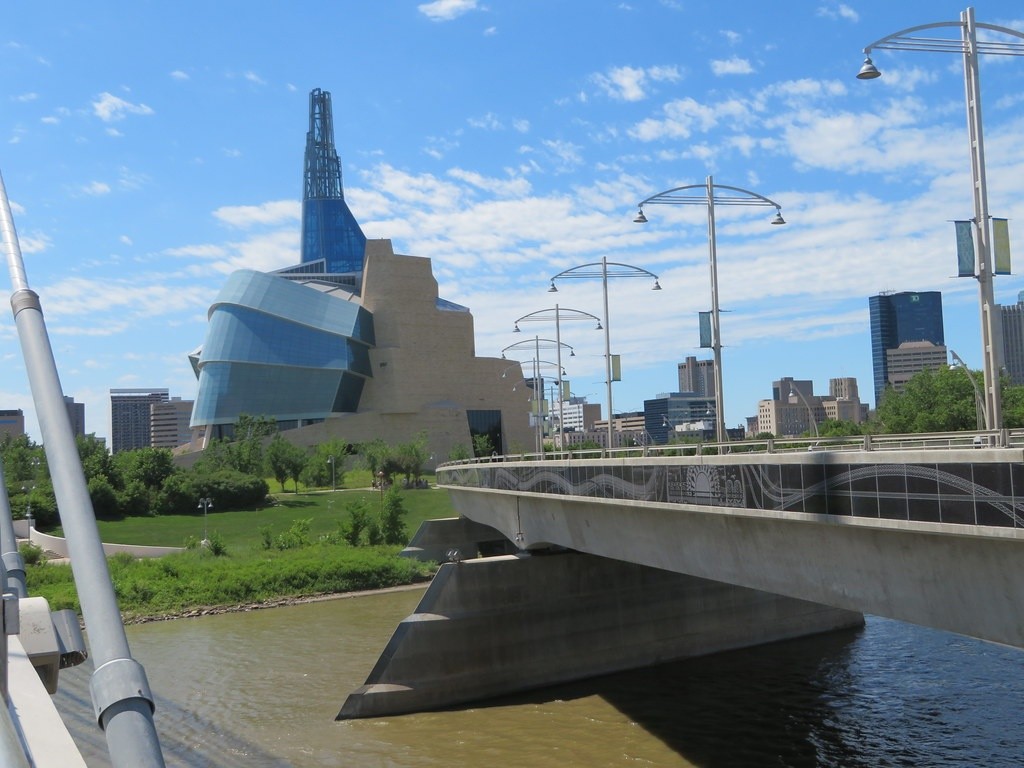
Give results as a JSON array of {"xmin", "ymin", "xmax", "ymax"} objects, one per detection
[
  {"xmin": 327, "ymin": 454, "xmax": 337, "ymax": 493},
  {"xmin": 377, "ymin": 470, "xmax": 385, "ymax": 520},
  {"xmin": 949, "ymin": 348, "xmax": 986, "ymax": 420},
  {"xmin": 854, "ymin": 6, "xmax": 1022, "ymax": 436},
  {"xmin": 788, "ymin": 380, "xmax": 820, "ymax": 438},
  {"xmin": 705, "ymin": 402, "xmax": 732, "ymax": 453},
  {"xmin": 500, "ymin": 303, "xmax": 601, "ymax": 460},
  {"xmin": 547, "ymin": 255, "xmax": 662, "ymax": 459},
  {"xmin": 198, "ymin": 497, "xmax": 215, "ymax": 540},
  {"xmin": 631, "ymin": 174, "xmax": 787, "ymax": 453}
]
